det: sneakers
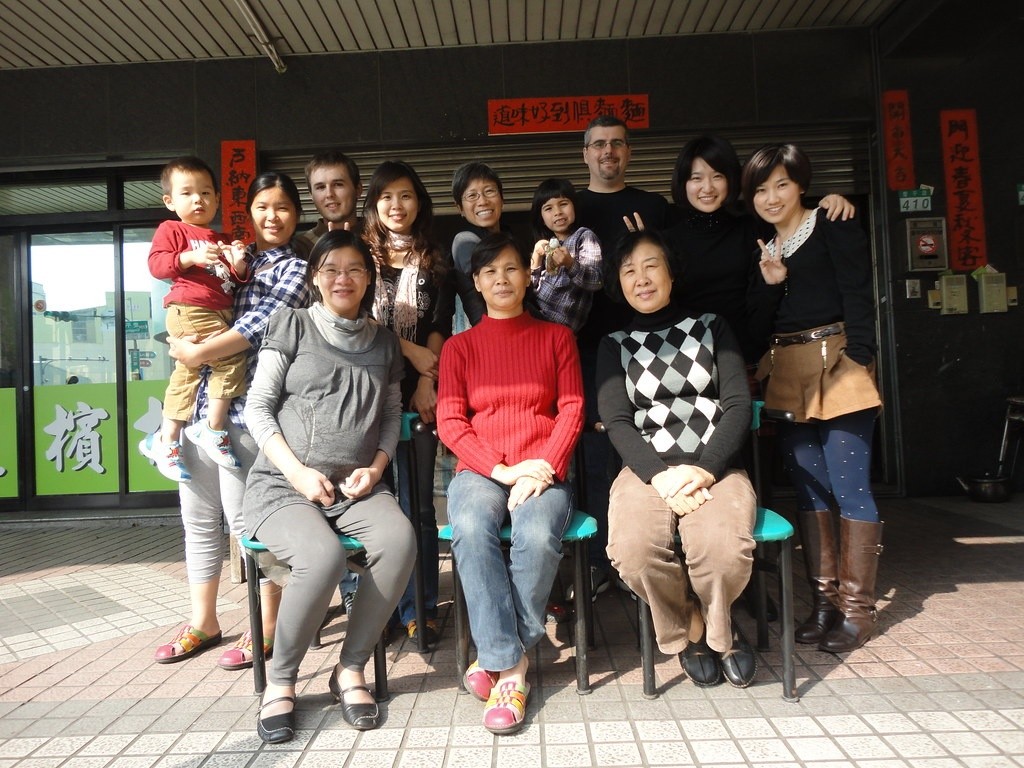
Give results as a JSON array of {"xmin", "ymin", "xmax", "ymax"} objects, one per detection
[
  {"xmin": 184, "ymin": 418, "xmax": 240, "ymax": 471},
  {"xmin": 139, "ymin": 434, "xmax": 192, "ymax": 484}
]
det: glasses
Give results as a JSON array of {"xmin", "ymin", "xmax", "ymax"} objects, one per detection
[
  {"xmin": 461, "ymin": 186, "xmax": 500, "ymax": 204},
  {"xmin": 584, "ymin": 140, "xmax": 628, "ymax": 151},
  {"xmin": 320, "ymin": 265, "xmax": 372, "ymax": 279}
]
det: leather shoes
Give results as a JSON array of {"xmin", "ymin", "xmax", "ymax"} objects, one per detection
[
  {"xmin": 329, "ymin": 662, "xmax": 382, "ymax": 728},
  {"xmin": 257, "ymin": 682, "xmax": 297, "ymax": 744}
]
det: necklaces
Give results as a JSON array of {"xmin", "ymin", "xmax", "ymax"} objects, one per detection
[{"xmin": 782, "ymin": 209, "xmax": 805, "ymax": 263}]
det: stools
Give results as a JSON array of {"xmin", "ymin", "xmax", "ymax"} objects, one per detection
[{"xmin": 993, "ymin": 394, "xmax": 1024, "ymax": 480}]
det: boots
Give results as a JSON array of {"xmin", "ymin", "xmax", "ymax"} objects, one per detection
[{"xmin": 795, "ymin": 510, "xmax": 884, "ymax": 653}]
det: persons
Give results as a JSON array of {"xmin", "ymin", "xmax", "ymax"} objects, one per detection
[
  {"xmin": 437, "ymin": 235, "xmax": 586, "ymax": 732},
  {"xmin": 622, "ymin": 138, "xmax": 854, "ymax": 622},
  {"xmin": 360, "ymin": 162, "xmax": 455, "ymax": 647},
  {"xmin": 154, "ymin": 174, "xmax": 314, "ymax": 670},
  {"xmin": 295, "ymin": 149, "xmax": 363, "ymax": 619},
  {"xmin": 525, "ymin": 179, "xmax": 603, "ymax": 335},
  {"xmin": 241, "ymin": 228, "xmax": 419, "ymax": 746},
  {"xmin": 741, "ymin": 144, "xmax": 884, "ymax": 653},
  {"xmin": 139, "ymin": 156, "xmax": 251, "ymax": 482},
  {"xmin": 567, "ymin": 117, "xmax": 673, "ymax": 602},
  {"xmin": 450, "ymin": 163, "xmax": 570, "ymax": 620},
  {"xmin": 597, "ymin": 228, "xmax": 758, "ymax": 687}
]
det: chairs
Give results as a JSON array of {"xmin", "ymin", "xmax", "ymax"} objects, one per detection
[
  {"xmin": 592, "ymin": 399, "xmax": 799, "ymax": 704},
  {"xmin": 236, "ymin": 412, "xmax": 418, "ymax": 702},
  {"xmin": 434, "ymin": 506, "xmax": 598, "ymax": 694}
]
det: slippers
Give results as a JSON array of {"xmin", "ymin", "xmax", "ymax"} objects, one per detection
[
  {"xmin": 220, "ymin": 630, "xmax": 273, "ymax": 670},
  {"xmin": 156, "ymin": 626, "xmax": 222, "ymax": 664},
  {"xmin": 462, "ymin": 658, "xmax": 531, "ymax": 734}
]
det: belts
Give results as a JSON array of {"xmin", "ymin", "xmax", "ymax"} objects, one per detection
[{"xmin": 773, "ymin": 326, "xmax": 843, "ymax": 348}]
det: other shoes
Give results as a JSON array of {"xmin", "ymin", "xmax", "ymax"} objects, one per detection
[
  {"xmin": 677, "ymin": 618, "xmax": 758, "ymax": 689},
  {"xmin": 615, "ymin": 575, "xmax": 636, "ymax": 601},
  {"xmin": 407, "ymin": 618, "xmax": 439, "ymax": 646},
  {"xmin": 546, "ymin": 601, "xmax": 568, "ymax": 624},
  {"xmin": 565, "ymin": 567, "xmax": 610, "ymax": 603}
]
